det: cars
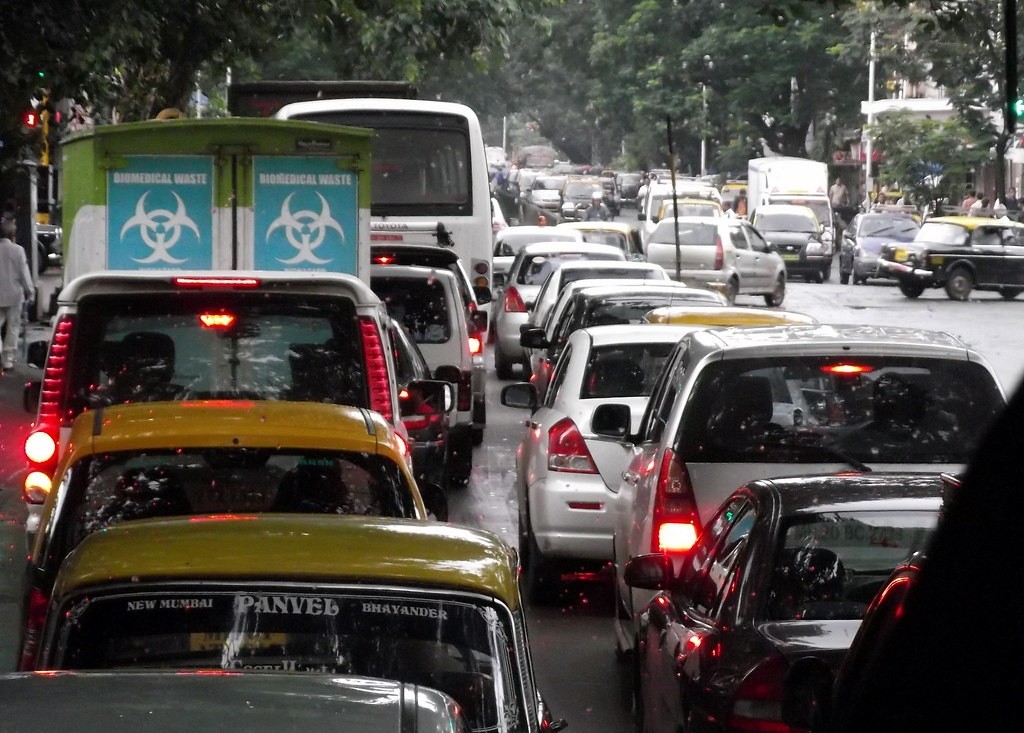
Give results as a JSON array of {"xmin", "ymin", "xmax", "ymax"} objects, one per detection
[
  {"xmin": 36, "ymin": 522, "xmax": 567, "ymax": 732},
  {"xmin": 280, "ymin": 79, "xmax": 833, "ymax": 323},
  {"xmin": 753, "ymin": 205, "xmax": 832, "ymax": 283},
  {"xmin": 842, "ymin": 212, "xmax": 915, "ymax": 286},
  {"xmin": 50, "ymin": 271, "xmax": 456, "ymax": 416},
  {"xmin": 591, "ymin": 329, "xmax": 1009, "ymax": 677},
  {"xmin": 627, "ymin": 474, "xmax": 986, "ymax": 733},
  {"xmin": 899, "ymin": 218, "xmax": 1023, "ymax": 298},
  {"xmin": 25, "ymin": 407, "xmax": 436, "ymax": 524},
  {"xmin": 500, "ymin": 328, "xmax": 688, "ymax": 598},
  {"xmin": 651, "ymin": 218, "xmax": 786, "ymax": 309}
]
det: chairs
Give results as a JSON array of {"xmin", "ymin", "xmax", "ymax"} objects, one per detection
[
  {"xmin": 268, "ymin": 464, "xmax": 347, "ymax": 514},
  {"xmin": 100, "ymin": 462, "xmax": 189, "ymax": 520},
  {"xmin": 593, "ymin": 359, "xmax": 645, "ymax": 395},
  {"xmin": 104, "ymin": 333, "xmax": 190, "ymax": 402},
  {"xmin": 776, "ymin": 549, "xmax": 851, "ymax": 618},
  {"xmin": 282, "ymin": 339, "xmax": 354, "ymax": 401},
  {"xmin": 706, "ymin": 375, "xmax": 788, "ymax": 442},
  {"xmin": 845, "ymin": 373, "xmax": 935, "ymax": 461}
]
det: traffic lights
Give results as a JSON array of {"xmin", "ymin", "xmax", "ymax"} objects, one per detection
[
  {"xmin": 1009, "ymin": 97, "xmax": 1024, "ymax": 117},
  {"xmin": 23, "ymin": 70, "xmax": 45, "ymax": 130}
]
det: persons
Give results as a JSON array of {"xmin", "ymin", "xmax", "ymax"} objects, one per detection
[
  {"xmin": 829, "ymin": 176, "xmax": 850, "ymax": 208},
  {"xmin": 0, "ymin": 220, "xmax": 36, "ymax": 370},
  {"xmin": 730, "ymin": 189, "xmax": 747, "ymax": 215},
  {"xmin": 1017, "ymin": 196, "xmax": 1024, "ymax": 211},
  {"xmin": 1007, "ymin": 187, "xmax": 1017, "ymax": 203},
  {"xmin": 495, "ymin": 168, "xmax": 504, "ymax": 184},
  {"xmin": 526, "ymin": 189, "xmax": 534, "ymax": 203},
  {"xmin": 963, "ymin": 190, "xmax": 976, "ymax": 209},
  {"xmin": 975, "ymin": 196, "xmax": 993, "ymax": 218},
  {"xmin": 877, "ymin": 192, "xmax": 886, "ymax": 204},
  {"xmin": 968, "ymin": 192, "xmax": 983, "ymax": 217},
  {"xmin": 508, "ymin": 165, "xmax": 520, "ymax": 183},
  {"xmin": 582, "ymin": 192, "xmax": 609, "ymax": 222},
  {"xmin": 638, "ymin": 177, "xmax": 651, "ymax": 214}
]
